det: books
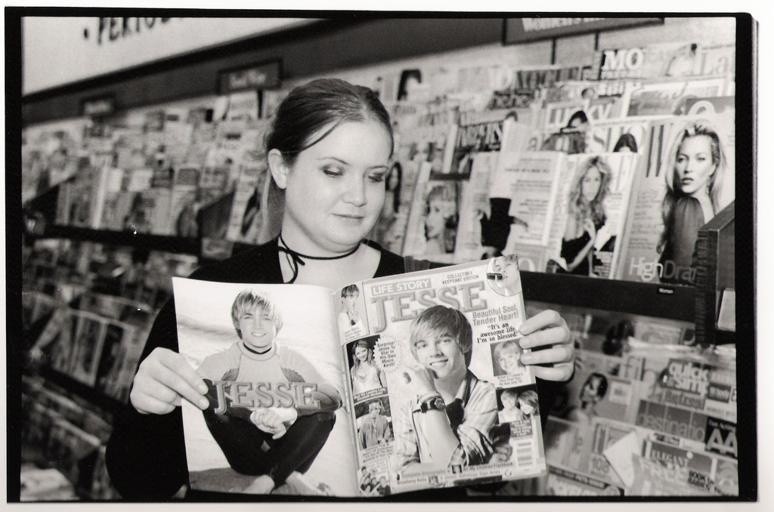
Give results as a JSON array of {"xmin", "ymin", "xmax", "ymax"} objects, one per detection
[{"xmin": 23, "ymin": 42, "xmax": 739, "ymax": 498}]
